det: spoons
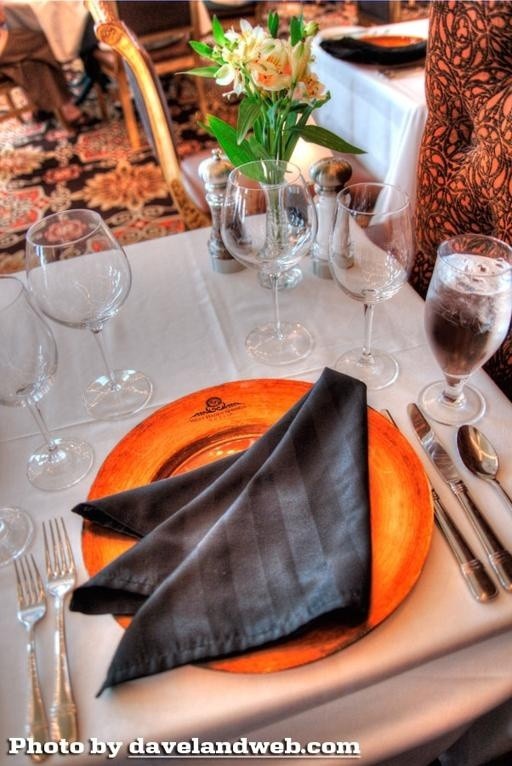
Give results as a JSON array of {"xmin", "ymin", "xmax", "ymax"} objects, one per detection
[{"xmin": 457, "ymin": 424, "xmax": 510, "ymax": 515}]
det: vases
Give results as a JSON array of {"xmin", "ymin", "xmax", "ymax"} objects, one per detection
[{"xmin": 256, "ymin": 179, "xmax": 302, "ymax": 290}]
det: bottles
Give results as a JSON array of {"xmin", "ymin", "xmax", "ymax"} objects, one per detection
[
  {"xmin": 194, "ymin": 147, "xmax": 244, "ymax": 271},
  {"xmin": 308, "ymin": 152, "xmax": 362, "ymax": 282}
]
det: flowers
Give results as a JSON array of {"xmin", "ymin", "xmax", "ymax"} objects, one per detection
[{"xmin": 171, "ymin": 12, "xmax": 368, "ymax": 252}]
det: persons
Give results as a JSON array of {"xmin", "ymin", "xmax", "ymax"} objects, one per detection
[
  {"xmin": 0, "ymin": 0, "xmax": 102, "ymax": 127},
  {"xmin": 67, "ymin": 2, "xmax": 109, "ymax": 106}
]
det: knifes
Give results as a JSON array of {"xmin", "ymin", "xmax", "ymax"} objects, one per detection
[{"xmin": 402, "ymin": 401, "xmax": 512, "ymax": 600}]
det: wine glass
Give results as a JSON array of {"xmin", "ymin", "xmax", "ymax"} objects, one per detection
[
  {"xmin": 222, "ymin": 161, "xmax": 318, "ymax": 364},
  {"xmin": 414, "ymin": 233, "xmax": 512, "ymax": 427},
  {"xmin": 0, "ymin": 278, "xmax": 93, "ymax": 492},
  {"xmin": 24, "ymin": 208, "xmax": 153, "ymax": 422},
  {"xmin": 329, "ymin": 179, "xmax": 406, "ymax": 390}
]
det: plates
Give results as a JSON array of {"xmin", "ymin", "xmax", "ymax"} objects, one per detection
[
  {"xmin": 359, "ymin": 35, "xmax": 424, "ymax": 49},
  {"xmin": 82, "ymin": 379, "xmax": 433, "ymax": 675}
]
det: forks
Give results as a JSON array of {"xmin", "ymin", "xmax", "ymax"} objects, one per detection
[{"xmin": 11, "ymin": 518, "xmax": 83, "ymax": 759}]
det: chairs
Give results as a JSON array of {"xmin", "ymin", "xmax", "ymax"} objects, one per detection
[{"xmin": 86, "ymin": 0, "xmax": 286, "ymax": 230}]
